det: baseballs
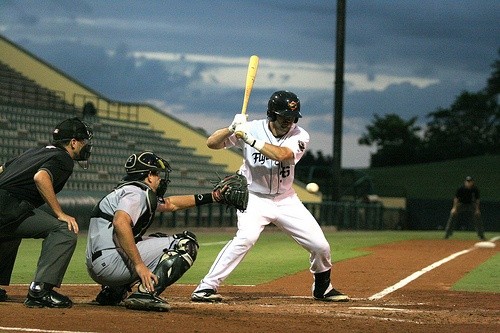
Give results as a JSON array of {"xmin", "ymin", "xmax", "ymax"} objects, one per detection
[{"xmin": 306, "ymin": 183, "xmax": 320, "ymax": 193}]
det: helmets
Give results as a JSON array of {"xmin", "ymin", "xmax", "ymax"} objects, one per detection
[
  {"xmin": 267, "ymin": 90, "xmax": 302, "ymax": 123},
  {"xmin": 126, "ymin": 151, "xmax": 172, "ymax": 173}
]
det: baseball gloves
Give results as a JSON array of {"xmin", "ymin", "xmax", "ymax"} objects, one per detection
[{"xmin": 211, "ymin": 171, "xmax": 249, "ymax": 213}]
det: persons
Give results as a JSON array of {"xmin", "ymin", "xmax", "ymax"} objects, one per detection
[
  {"xmin": 86, "ymin": 152, "xmax": 227, "ymax": 311},
  {"xmin": 0, "ymin": 117, "xmax": 94, "ymax": 308},
  {"xmin": 191, "ymin": 91, "xmax": 351, "ymax": 302},
  {"xmin": 443, "ymin": 176, "xmax": 488, "ymax": 241}
]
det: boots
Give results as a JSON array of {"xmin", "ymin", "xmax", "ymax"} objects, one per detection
[
  {"xmin": 313, "ymin": 269, "xmax": 350, "ymax": 301},
  {"xmin": 191, "ymin": 289, "xmax": 222, "ymax": 302}
]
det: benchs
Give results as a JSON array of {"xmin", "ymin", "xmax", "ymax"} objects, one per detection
[{"xmin": 1, "ymin": 60, "xmax": 236, "ymax": 196}]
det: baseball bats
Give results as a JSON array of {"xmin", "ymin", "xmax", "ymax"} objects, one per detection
[{"xmin": 234, "ymin": 54, "xmax": 259, "ymax": 141}]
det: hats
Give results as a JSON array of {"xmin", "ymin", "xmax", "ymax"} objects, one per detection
[
  {"xmin": 466, "ymin": 176, "xmax": 472, "ymax": 181},
  {"xmin": 53, "ymin": 117, "xmax": 94, "ymax": 140}
]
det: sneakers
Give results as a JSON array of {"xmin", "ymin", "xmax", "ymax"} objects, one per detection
[
  {"xmin": 213, "ymin": 174, "xmax": 249, "ymax": 210},
  {"xmin": 0, "ymin": 288, "xmax": 8, "ymax": 301},
  {"xmin": 125, "ymin": 290, "xmax": 171, "ymax": 312},
  {"xmin": 24, "ymin": 282, "xmax": 73, "ymax": 309},
  {"xmin": 96, "ymin": 289, "xmax": 128, "ymax": 306}
]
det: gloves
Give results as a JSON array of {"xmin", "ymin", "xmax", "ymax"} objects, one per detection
[
  {"xmin": 228, "ymin": 113, "xmax": 248, "ymax": 132},
  {"xmin": 234, "ymin": 123, "xmax": 265, "ymax": 151}
]
